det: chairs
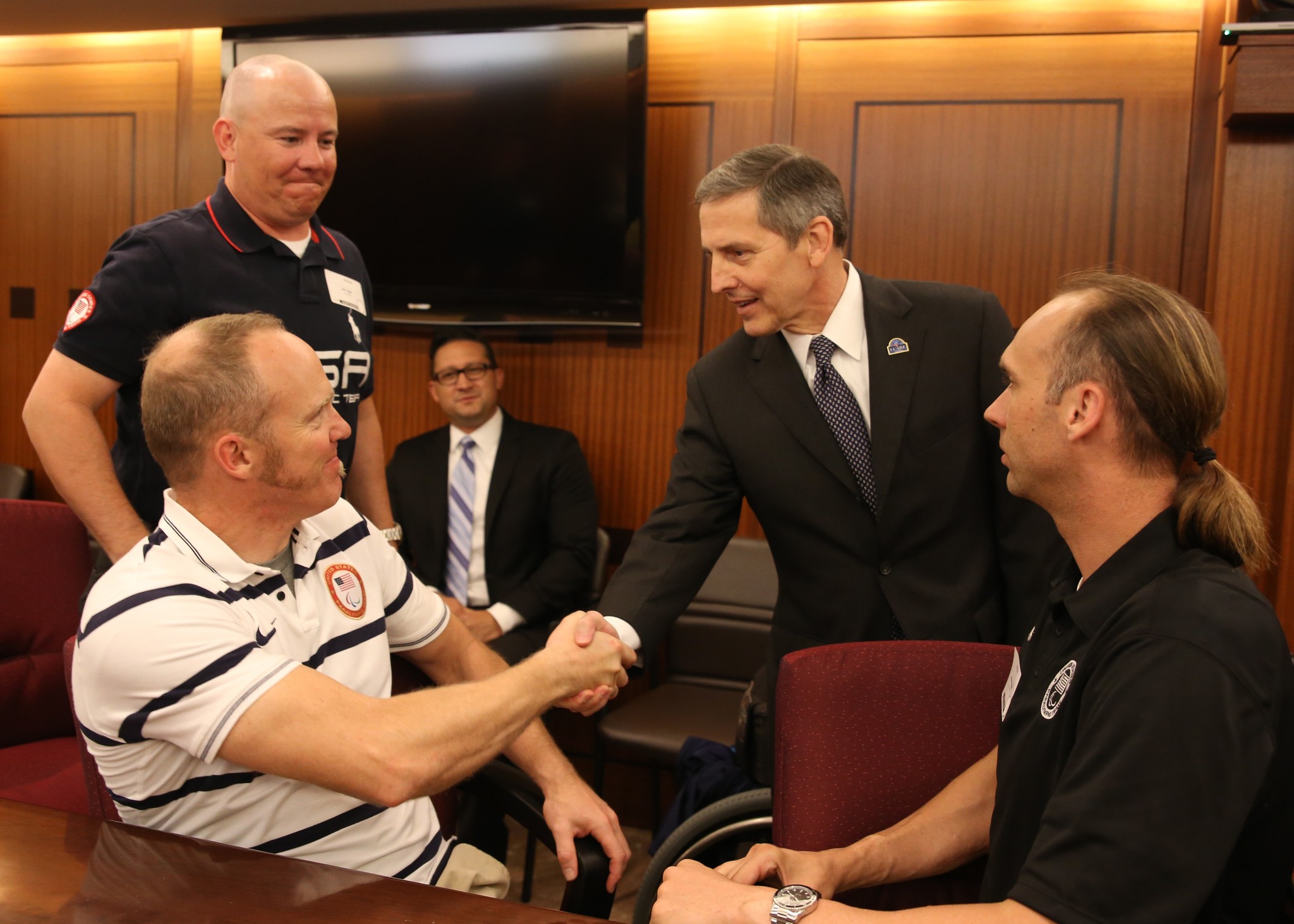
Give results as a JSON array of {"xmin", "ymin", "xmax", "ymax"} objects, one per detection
[
  {"xmin": 0, "ymin": 497, "xmax": 616, "ymax": 919},
  {"xmin": 590, "ymin": 538, "xmax": 778, "ymax": 838},
  {"xmin": 633, "ymin": 638, "xmax": 1022, "ymax": 924}
]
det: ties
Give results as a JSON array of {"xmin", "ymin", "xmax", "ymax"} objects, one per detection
[
  {"xmin": 446, "ymin": 437, "xmax": 477, "ymax": 606},
  {"xmin": 809, "ymin": 334, "xmax": 876, "ymax": 516}
]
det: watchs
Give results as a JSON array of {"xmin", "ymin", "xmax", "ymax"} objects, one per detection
[
  {"xmin": 379, "ymin": 523, "xmax": 403, "ymax": 542},
  {"xmin": 769, "ymin": 886, "xmax": 822, "ymax": 924}
]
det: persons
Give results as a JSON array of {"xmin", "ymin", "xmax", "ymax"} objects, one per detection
[
  {"xmin": 69, "ymin": 317, "xmax": 637, "ymax": 900},
  {"xmin": 648, "ymin": 275, "xmax": 1293, "ymax": 924},
  {"xmin": 542, "ymin": 145, "xmax": 1083, "ymax": 796},
  {"xmin": 21, "ymin": 56, "xmax": 403, "ymax": 570},
  {"xmin": 384, "ymin": 330, "xmax": 599, "ymax": 867}
]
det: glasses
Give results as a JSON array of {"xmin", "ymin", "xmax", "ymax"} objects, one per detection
[{"xmin": 429, "ymin": 362, "xmax": 495, "ymax": 385}]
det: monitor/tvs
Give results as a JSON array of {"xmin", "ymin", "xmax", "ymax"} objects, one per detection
[{"xmin": 218, "ymin": 8, "xmax": 648, "ymax": 345}]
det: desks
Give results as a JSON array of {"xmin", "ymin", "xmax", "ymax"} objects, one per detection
[{"xmin": 0, "ymin": 798, "xmax": 622, "ymax": 924}]
